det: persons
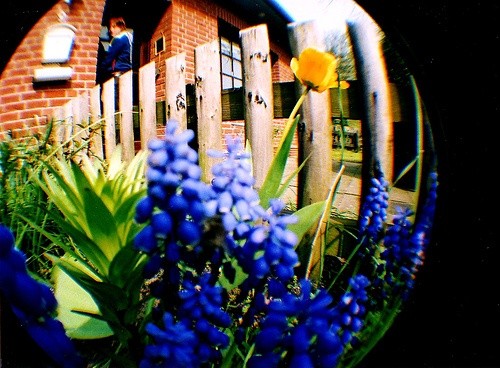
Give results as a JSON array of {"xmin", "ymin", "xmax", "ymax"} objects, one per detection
[{"xmin": 102, "ymin": 15, "xmax": 131, "ymax": 79}]
